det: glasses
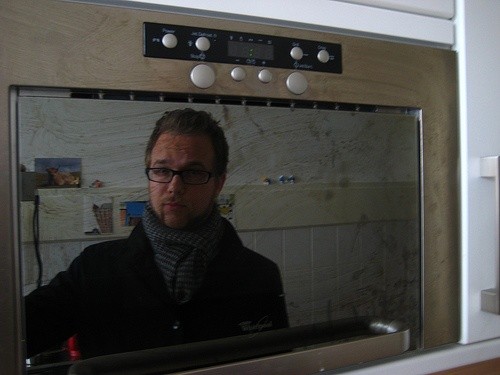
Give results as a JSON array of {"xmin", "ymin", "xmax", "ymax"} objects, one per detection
[{"xmin": 145, "ymin": 167, "xmax": 218, "ymax": 185}]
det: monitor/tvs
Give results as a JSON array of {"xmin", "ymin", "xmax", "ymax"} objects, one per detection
[{"xmin": 7, "ymin": 84, "xmax": 426, "ymax": 375}]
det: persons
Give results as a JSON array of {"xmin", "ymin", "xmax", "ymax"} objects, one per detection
[{"xmin": 21, "ymin": 105, "xmax": 295, "ymax": 374}]
